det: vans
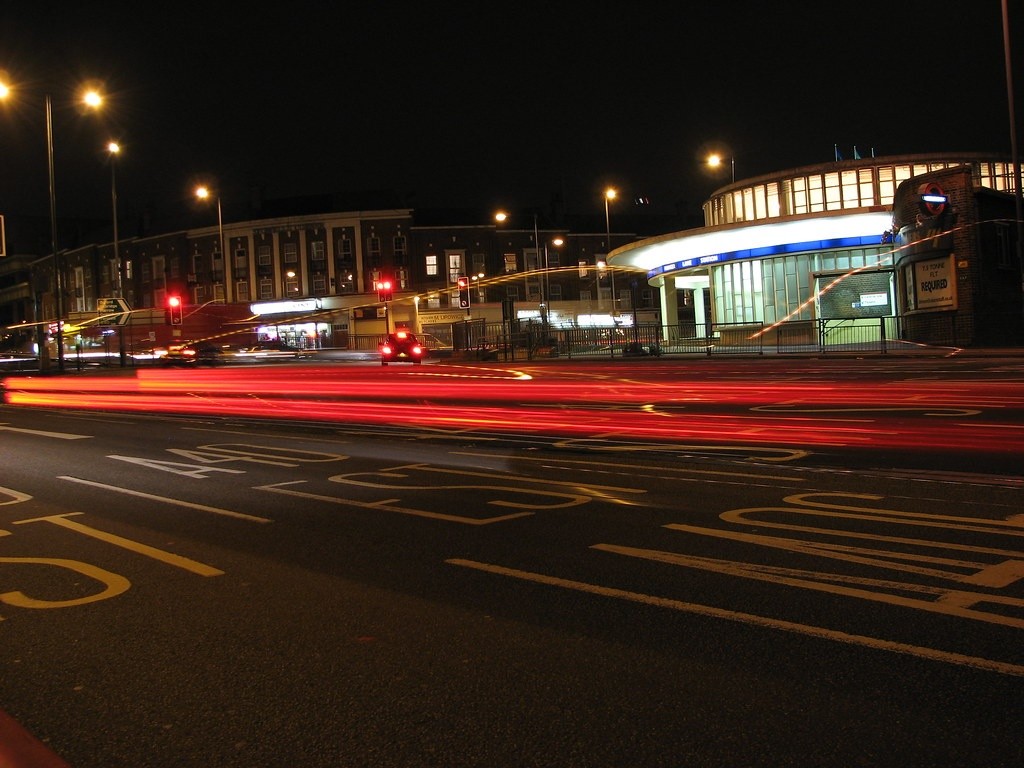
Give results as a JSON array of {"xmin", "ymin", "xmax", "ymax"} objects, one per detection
[{"xmin": 415, "ymin": 334, "xmax": 448, "ymax": 349}]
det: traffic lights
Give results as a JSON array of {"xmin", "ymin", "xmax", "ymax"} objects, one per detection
[
  {"xmin": 384, "ymin": 281, "xmax": 392, "ymax": 301},
  {"xmin": 457, "ymin": 277, "xmax": 471, "ymax": 310},
  {"xmin": 377, "ymin": 283, "xmax": 384, "ymax": 302},
  {"xmin": 167, "ymin": 296, "xmax": 182, "ymax": 325}
]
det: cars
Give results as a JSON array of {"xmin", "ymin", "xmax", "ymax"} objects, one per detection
[
  {"xmin": 168, "ymin": 339, "xmax": 193, "ymax": 356},
  {"xmin": 170, "ymin": 342, "xmax": 225, "ymax": 363},
  {"xmin": 378, "ymin": 333, "xmax": 423, "ymax": 366}
]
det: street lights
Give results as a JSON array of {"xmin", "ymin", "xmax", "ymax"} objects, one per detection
[
  {"xmin": 544, "ymin": 237, "xmax": 563, "ymax": 324},
  {"xmin": 496, "ymin": 209, "xmax": 549, "ymax": 320},
  {"xmin": 197, "ymin": 187, "xmax": 230, "ymax": 301},
  {"xmin": 109, "ymin": 140, "xmax": 136, "ymax": 367},
  {"xmin": 596, "ymin": 185, "xmax": 620, "ymax": 324},
  {"xmin": 40, "ymin": 79, "xmax": 107, "ymax": 369},
  {"xmin": 0, "ymin": 76, "xmax": 10, "ymax": 105},
  {"xmin": 709, "ymin": 155, "xmax": 735, "ymax": 182}
]
